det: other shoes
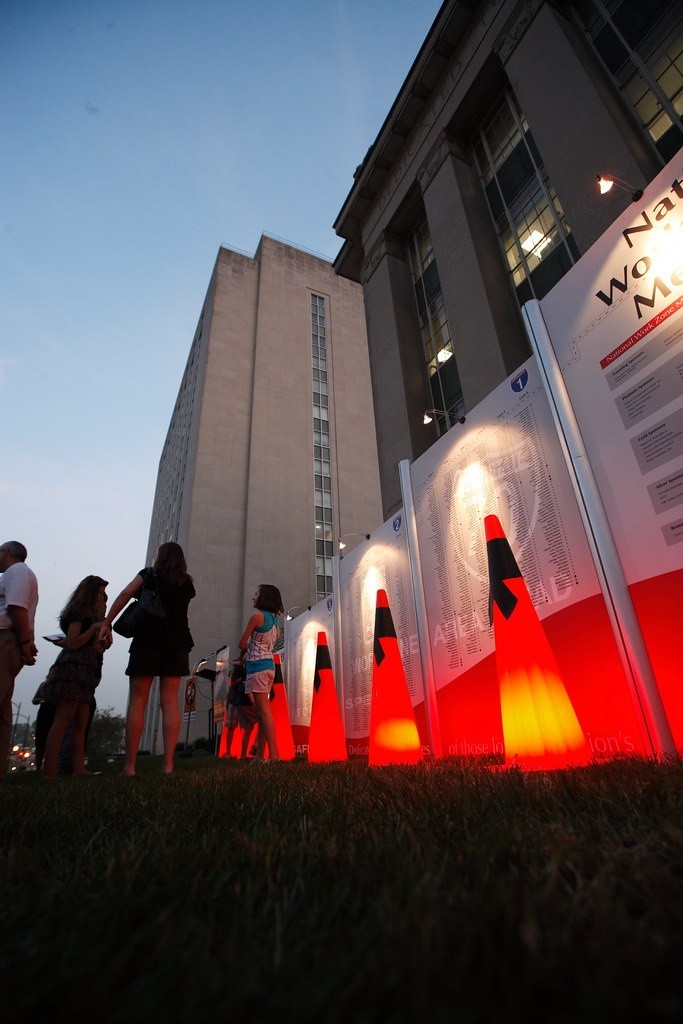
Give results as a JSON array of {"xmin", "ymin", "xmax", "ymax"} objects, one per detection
[{"xmin": 249, "ymin": 755, "xmax": 281, "ymax": 768}]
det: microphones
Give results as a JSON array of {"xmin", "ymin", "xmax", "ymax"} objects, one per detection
[{"xmin": 212, "ymin": 646, "xmax": 228, "ymax": 655}]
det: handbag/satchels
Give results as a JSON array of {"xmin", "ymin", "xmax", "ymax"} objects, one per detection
[{"xmin": 112, "ymin": 591, "xmax": 166, "ymax": 639}]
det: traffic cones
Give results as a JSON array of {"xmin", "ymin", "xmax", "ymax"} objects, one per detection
[
  {"xmin": 263, "ymin": 653, "xmax": 295, "ymax": 761},
  {"xmin": 473, "ymin": 515, "xmax": 626, "ymax": 771},
  {"xmin": 307, "ymin": 631, "xmax": 348, "ymax": 764},
  {"xmin": 219, "ymin": 678, "xmax": 258, "ymax": 759},
  {"xmin": 366, "ymin": 588, "xmax": 424, "ymax": 767}
]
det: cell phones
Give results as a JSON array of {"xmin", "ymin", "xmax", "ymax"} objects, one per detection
[{"xmin": 103, "ymin": 634, "xmax": 111, "ymax": 649}]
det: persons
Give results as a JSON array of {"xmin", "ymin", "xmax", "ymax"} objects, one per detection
[
  {"xmin": 223, "ymin": 583, "xmax": 286, "ymax": 766},
  {"xmin": 0, "ymin": 540, "xmax": 39, "ymax": 774},
  {"xmin": 33, "ymin": 542, "xmax": 197, "ymax": 782}
]
